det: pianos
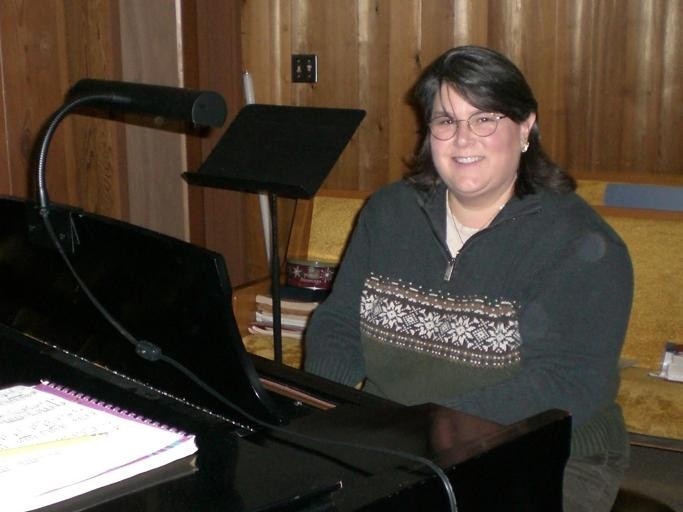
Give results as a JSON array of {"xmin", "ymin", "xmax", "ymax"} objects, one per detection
[{"xmin": 0, "ymin": 191, "xmax": 570, "ymax": 512}]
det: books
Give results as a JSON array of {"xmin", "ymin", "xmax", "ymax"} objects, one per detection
[
  {"xmin": 647, "ymin": 341, "xmax": 683, "ymax": 383},
  {"xmin": 0, "ymin": 379, "xmax": 199, "ymax": 512},
  {"xmin": 246, "ymin": 283, "xmax": 323, "ymax": 342}
]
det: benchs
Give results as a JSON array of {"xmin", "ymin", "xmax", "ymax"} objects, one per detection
[{"xmin": 232, "ymin": 189, "xmax": 683, "ymax": 512}]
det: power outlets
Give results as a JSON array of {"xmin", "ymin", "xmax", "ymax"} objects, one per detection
[{"xmin": 292, "ymin": 54, "xmax": 318, "ymax": 83}]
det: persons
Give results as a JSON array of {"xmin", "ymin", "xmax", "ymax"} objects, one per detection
[{"xmin": 301, "ymin": 44, "xmax": 635, "ymax": 511}]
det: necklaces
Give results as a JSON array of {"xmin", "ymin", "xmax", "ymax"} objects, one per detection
[{"xmin": 444, "ymin": 185, "xmax": 513, "ymax": 247}]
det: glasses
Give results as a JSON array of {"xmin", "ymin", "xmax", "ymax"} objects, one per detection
[{"xmin": 425, "ymin": 112, "xmax": 507, "ymax": 141}]
